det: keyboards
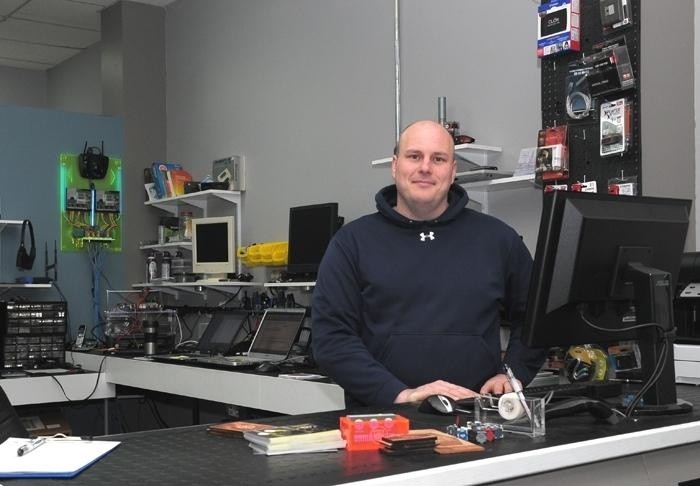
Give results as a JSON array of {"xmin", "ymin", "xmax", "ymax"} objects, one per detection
[{"xmin": 454, "ymin": 379, "xmax": 590, "ymax": 410}]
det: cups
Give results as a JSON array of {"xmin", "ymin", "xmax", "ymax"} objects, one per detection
[{"xmin": 142, "ymin": 320, "xmax": 159, "ymax": 355}]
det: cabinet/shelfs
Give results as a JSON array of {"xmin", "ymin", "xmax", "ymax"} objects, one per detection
[
  {"xmin": 132, "ymin": 189, "xmax": 317, "ymax": 288},
  {"xmin": 0, "ymin": 220, "xmax": 51, "ymax": 288},
  {"xmin": 371, "ymin": 143, "xmax": 536, "ymax": 193}
]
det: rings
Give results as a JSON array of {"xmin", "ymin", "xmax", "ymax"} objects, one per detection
[{"xmin": 504, "ymin": 380, "xmax": 510, "ymax": 384}]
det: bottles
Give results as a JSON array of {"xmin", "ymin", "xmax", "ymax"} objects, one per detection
[
  {"xmin": 179, "ymin": 212, "xmax": 192, "ymax": 242},
  {"xmin": 145, "ymin": 256, "xmax": 158, "ymax": 283},
  {"xmin": 161, "ymin": 256, "xmax": 170, "ymax": 280}
]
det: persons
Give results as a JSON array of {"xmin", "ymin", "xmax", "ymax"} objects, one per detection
[{"xmin": 310, "ymin": 119, "xmax": 550, "ymax": 410}]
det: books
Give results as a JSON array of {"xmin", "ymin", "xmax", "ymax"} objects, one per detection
[
  {"xmin": 243, "ymin": 421, "xmax": 347, "ymax": 456},
  {"xmin": 210, "ymin": 421, "xmax": 272, "ymax": 438}
]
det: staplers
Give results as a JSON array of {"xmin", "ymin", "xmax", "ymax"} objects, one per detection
[{"xmin": 379, "ymin": 433, "xmax": 438, "ymax": 456}]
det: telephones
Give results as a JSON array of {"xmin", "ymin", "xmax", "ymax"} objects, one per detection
[
  {"xmin": 75, "ymin": 320, "xmax": 87, "ymax": 348},
  {"xmin": 40, "ymin": 357, "xmax": 58, "ymax": 369}
]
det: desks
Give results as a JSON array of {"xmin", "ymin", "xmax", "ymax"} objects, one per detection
[
  {"xmin": 0, "ymin": 381, "xmax": 700, "ymax": 486},
  {"xmin": 0, "ymin": 351, "xmax": 346, "ymax": 434}
]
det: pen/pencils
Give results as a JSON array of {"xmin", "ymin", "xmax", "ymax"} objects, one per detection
[
  {"xmin": 503, "ymin": 362, "xmax": 540, "ymax": 428},
  {"xmin": 17, "ymin": 438, "xmax": 47, "ymax": 456},
  {"xmin": 195, "ymin": 279, "xmax": 231, "ymax": 282}
]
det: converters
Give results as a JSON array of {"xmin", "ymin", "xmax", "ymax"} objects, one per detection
[{"xmin": 581, "ymin": 379, "xmax": 623, "ymax": 398}]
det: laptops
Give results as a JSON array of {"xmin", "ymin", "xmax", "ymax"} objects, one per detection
[
  {"xmin": 144, "ymin": 309, "xmax": 249, "ymax": 363},
  {"xmin": 185, "ymin": 307, "xmax": 307, "ymax": 365}
]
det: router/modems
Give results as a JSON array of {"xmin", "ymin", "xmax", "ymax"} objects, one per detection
[{"xmin": 79, "ymin": 138, "xmax": 110, "ymax": 180}]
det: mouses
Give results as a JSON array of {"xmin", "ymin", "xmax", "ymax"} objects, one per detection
[
  {"xmin": 255, "ymin": 362, "xmax": 282, "ymax": 375},
  {"xmin": 418, "ymin": 394, "xmax": 456, "ymax": 415}
]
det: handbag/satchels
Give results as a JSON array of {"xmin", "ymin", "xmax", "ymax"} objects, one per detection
[{"xmin": 16, "ymin": 243, "xmax": 36, "ymax": 270}]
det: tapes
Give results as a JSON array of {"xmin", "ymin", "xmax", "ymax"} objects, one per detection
[{"xmin": 498, "ymin": 393, "xmax": 523, "ymax": 421}]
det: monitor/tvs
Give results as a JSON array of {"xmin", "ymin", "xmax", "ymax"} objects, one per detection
[
  {"xmin": 286, "ymin": 202, "xmax": 340, "ymax": 276},
  {"xmin": 190, "ymin": 216, "xmax": 236, "ymax": 278},
  {"xmin": 522, "ymin": 188, "xmax": 700, "ymax": 415}
]
